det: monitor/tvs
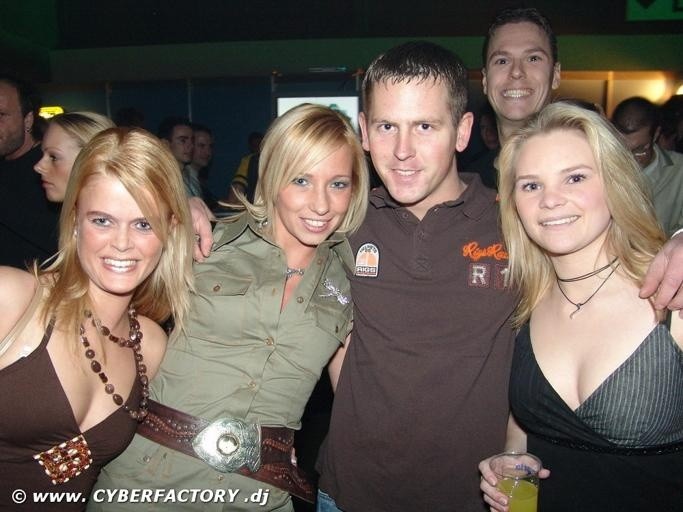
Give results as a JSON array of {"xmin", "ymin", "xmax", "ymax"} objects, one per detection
[{"xmin": 271, "ymin": 88, "xmax": 371, "ymax": 156}]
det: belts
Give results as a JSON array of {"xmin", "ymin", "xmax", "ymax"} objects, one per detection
[{"xmin": 135, "ymin": 398, "xmax": 320, "ymax": 506}]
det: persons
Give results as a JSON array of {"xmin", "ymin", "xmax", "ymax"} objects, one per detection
[
  {"xmin": 32, "ymin": 111, "xmax": 115, "ymax": 202},
  {"xmin": 0, "ymin": 76, "xmax": 67, "ymax": 273},
  {"xmin": 188, "ymin": 40, "xmax": 683, "ymax": 512},
  {"xmin": 0, "ymin": 127, "xmax": 195, "ymax": 512},
  {"xmin": 496, "ymin": 103, "xmax": 683, "ymax": 512},
  {"xmin": 109, "ymin": 103, "xmax": 264, "ymax": 220},
  {"xmin": 84, "ymin": 104, "xmax": 371, "ymax": 512}
]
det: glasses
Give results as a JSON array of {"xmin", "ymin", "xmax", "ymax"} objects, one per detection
[{"xmin": 632, "ymin": 140, "xmax": 652, "ymax": 158}]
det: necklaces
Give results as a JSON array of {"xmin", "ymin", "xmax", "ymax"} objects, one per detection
[
  {"xmin": 553, "ymin": 255, "xmax": 621, "ymax": 319},
  {"xmin": 77, "ymin": 305, "xmax": 149, "ymax": 422},
  {"xmin": 286, "ymin": 267, "xmax": 307, "ymax": 281}
]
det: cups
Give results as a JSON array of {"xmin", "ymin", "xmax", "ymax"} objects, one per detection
[{"xmin": 485, "ymin": 452, "xmax": 542, "ymax": 511}]
